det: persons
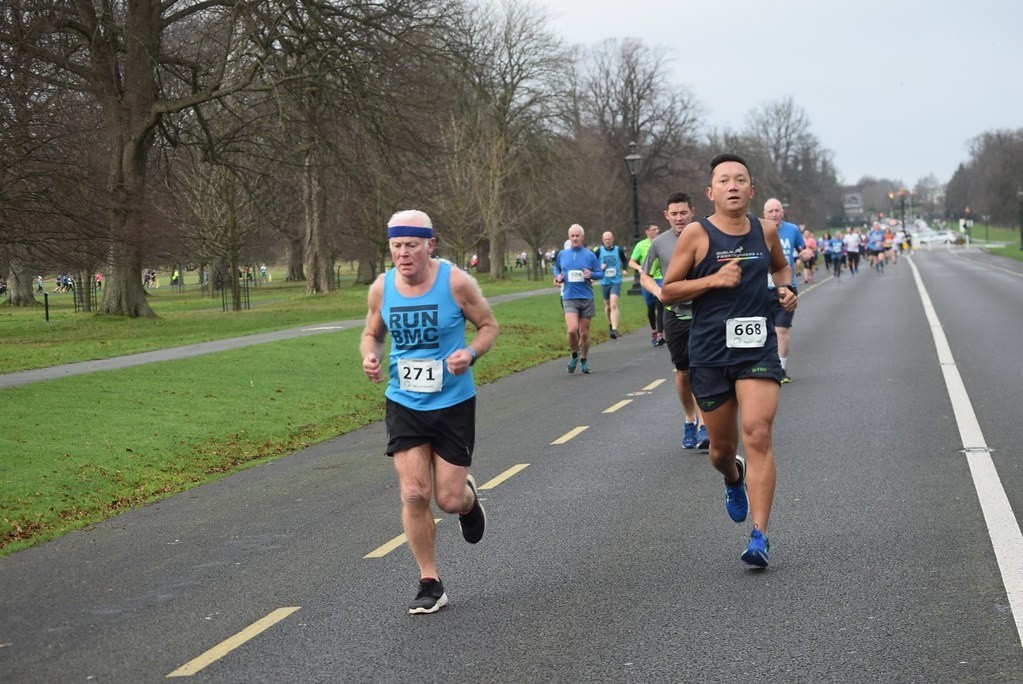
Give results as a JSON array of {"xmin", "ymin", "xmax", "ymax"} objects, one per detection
[
  {"xmin": 360, "ymin": 210, "xmax": 500, "ymax": 613},
  {"xmin": 245, "ymin": 263, "xmax": 266, "ymax": 281},
  {"xmin": 763, "ymin": 198, "xmax": 810, "ymax": 383},
  {"xmin": 553, "ymin": 224, "xmax": 603, "ymax": 373},
  {"xmin": 96, "ymin": 272, "xmax": 102, "ymax": 290},
  {"xmin": 793, "ymin": 221, "xmax": 905, "ymax": 282},
  {"xmin": 593, "ymin": 231, "xmax": 628, "ymax": 339},
  {"xmin": 143, "ymin": 269, "xmax": 156, "ymax": 287},
  {"xmin": 515, "ymin": 250, "xmax": 527, "ymax": 269},
  {"xmin": 538, "ymin": 248, "xmax": 555, "ymax": 268},
  {"xmin": 37, "ymin": 276, "xmax": 43, "ymax": 293},
  {"xmin": 660, "ymin": 154, "xmax": 798, "ymax": 568},
  {"xmin": 472, "ymin": 252, "xmax": 477, "ymax": 267},
  {"xmin": 629, "ymin": 222, "xmax": 665, "ymax": 347},
  {"xmin": 53, "ymin": 273, "xmax": 74, "ymax": 293},
  {"xmin": 640, "ymin": 193, "xmax": 710, "ymax": 449}
]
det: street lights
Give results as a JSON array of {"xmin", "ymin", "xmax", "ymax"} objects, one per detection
[
  {"xmin": 1016, "ymin": 186, "xmax": 1023, "ymax": 250},
  {"xmin": 625, "ymin": 141, "xmax": 642, "ymax": 295}
]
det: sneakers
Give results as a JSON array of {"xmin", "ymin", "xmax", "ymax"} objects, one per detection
[
  {"xmin": 460, "ymin": 474, "xmax": 486, "ymax": 545},
  {"xmin": 741, "ymin": 523, "xmax": 770, "ymax": 568},
  {"xmin": 781, "ymin": 368, "xmax": 792, "ymax": 383},
  {"xmin": 610, "ymin": 332, "xmax": 617, "ymax": 339},
  {"xmin": 651, "ymin": 332, "xmax": 658, "ymax": 346},
  {"xmin": 656, "ymin": 334, "xmax": 665, "ymax": 346},
  {"xmin": 579, "ymin": 361, "xmax": 591, "ymax": 373},
  {"xmin": 409, "ymin": 577, "xmax": 448, "ymax": 614},
  {"xmin": 695, "ymin": 425, "xmax": 711, "ymax": 450},
  {"xmin": 723, "ymin": 454, "xmax": 750, "ymax": 523},
  {"xmin": 567, "ymin": 356, "xmax": 578, "ymax": 373},
  {"xmin": 681, "ymin": 418, "xmax": 698, "ymax": 450}
]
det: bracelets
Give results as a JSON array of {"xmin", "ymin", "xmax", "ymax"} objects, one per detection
[
  {"xmin": 780, "ymin": 284, "xmax": 796, "ymax": 295},
  {"xmin": 467, "ymin": 348, "xmax": 477, "ymax": 366}
]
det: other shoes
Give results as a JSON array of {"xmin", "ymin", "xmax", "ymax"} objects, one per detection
[{"xmin": 795, "ymin": 263, "xmax": 898, "ymax": 285}]
